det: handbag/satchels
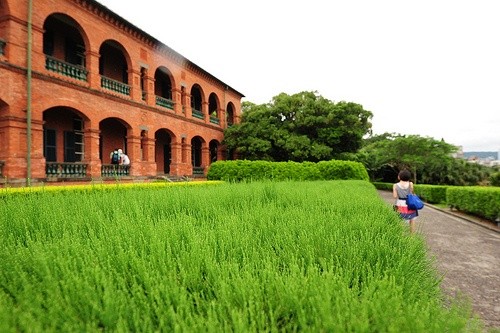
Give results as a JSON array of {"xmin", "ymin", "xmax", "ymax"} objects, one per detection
[{"xmin": 406, "ymin": 193, "xmax": 424, "ymax": 210}]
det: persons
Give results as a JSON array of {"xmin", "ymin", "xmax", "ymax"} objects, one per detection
[
  {"xmin": 393, "ymin": 170, "xmax": 417, "ymax": 234},
  {"xmin": 119, "ymin": 153, "xmax": 130, "ymax": 175},
  {"xmin": 110, "ymin": 149, "xmax": 121, "ymax": 169}
]
